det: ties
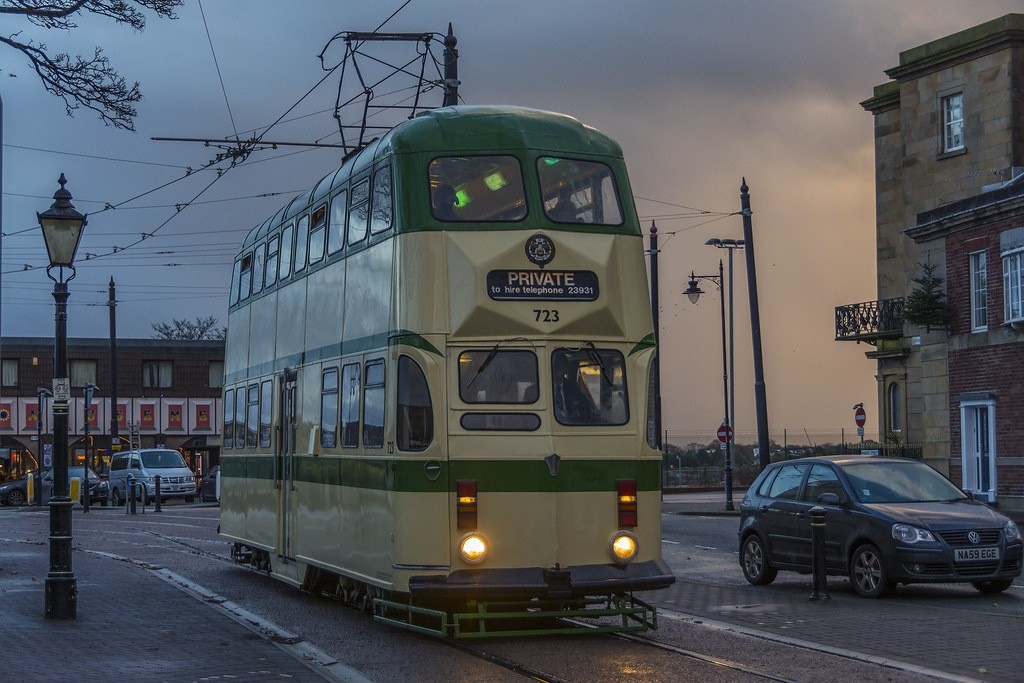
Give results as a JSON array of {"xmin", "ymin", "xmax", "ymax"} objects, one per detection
[{"xmin": 556, "ymin": 384, "xmax": 563, "ymax": 412}]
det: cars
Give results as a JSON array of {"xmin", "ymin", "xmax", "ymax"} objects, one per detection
[
  {"xmin": 40, "ymin": 466, "xmax": 110, "ymax": 507},
  {"xmin": 737, "ymin": 454, "xmax": 1024, "ymax": 601},
  {"xmin": 0, "ymin": 466, "xmax": 50, "ymax": 507},
  {"xmin": 200, "ymin": 464, "xmax": 221, "ymax": 504}
]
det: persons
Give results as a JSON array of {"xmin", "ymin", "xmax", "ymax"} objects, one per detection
[
  {"xmin": 523, "ymin": 352, "xmax": 599, "ymax": 423},
  {"xmin": 432, "ymin": 184, "xmax": 462, "ymax": 219},
  {"xmin": 459, "ymin": 178, "xmax": 500, "ymax": 219},
  {"xmin": 548, "ymin": 187, "xmax": 577, "ymax": 222}
]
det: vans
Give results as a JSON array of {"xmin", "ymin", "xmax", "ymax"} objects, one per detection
[{"xmin": 108, "ymin": 448, "xmax": 198, "ymax": 507}]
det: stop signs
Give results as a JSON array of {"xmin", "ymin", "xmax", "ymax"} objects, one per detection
[
  {"xmin": 717, "ymin": 426, "xmax": 735, "ymax": 444},
  {"xmin": 855, "ymin": 407, "xmax": 867, "ymax": 427}
]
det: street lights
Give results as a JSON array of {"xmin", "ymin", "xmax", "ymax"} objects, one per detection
[
  {"xmin": 684, "ymin": 258, "xmax": 740, "ymax": 513},
  {"xmin": 704, "ymin": 234, "xmax": 747, "ymax": 441},
  {"xmin": 36, "ymin": 172, "xmax": 86, "ymax": 625}
]
